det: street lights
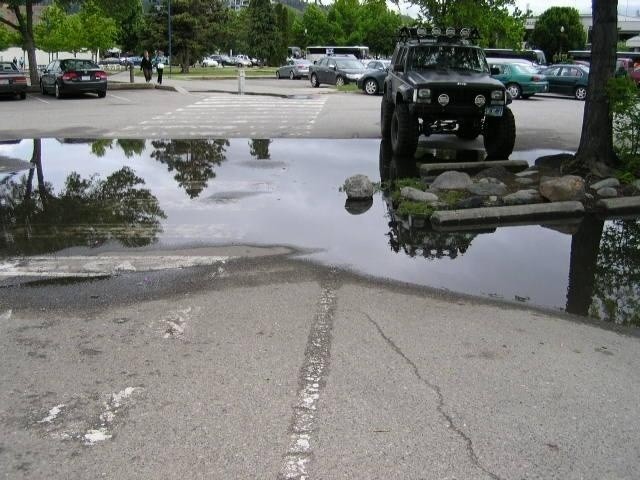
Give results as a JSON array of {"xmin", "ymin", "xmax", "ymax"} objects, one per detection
[
  {"xmin": 304, "ymin": 28, "xmax": 308, "ymax": 51},
  {"xmin": 153, "ymin": 0, "xmax": 172, "ymax": 73},
  {"xmin": 560, "ymin": 25, "xmax": 565, "ymax": 54}
]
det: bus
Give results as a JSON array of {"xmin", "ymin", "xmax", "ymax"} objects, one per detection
[
  {"xmin": 482, "ymin": 48, "xmax": 548, "ymax": 68},
  {"xmin": 305, "ymin": 45, "xmax": 371, "ymax": 60},
  {"xmin": 287, "ymin": 46, "xmax": 303, "ymax": 59}
]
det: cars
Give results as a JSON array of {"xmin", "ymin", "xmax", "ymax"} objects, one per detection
[
  {"xmin": 308, "ymin": 56, "xmax": 365, "ymax": 87},
  {"xmin": 357, "ymin": 58, "xmax": 391, "ymax": 96},
  {"xmin": 275, "ymin": 58, "xmax": 315, "ymax": 80},
  {"xmin": 0, "ymin": 61, "xmax": 32, "ymax": 100},
  {"xmin": 484, "ymin": 57, "xmax": 549, "ymax": 101},
  {"xmin": 97, "ymin": 53, "xmax": 268, "ymax": 68},
  {"xmin": 537, "ymin": 50, "xmax": 640, "ymax": 102},
  {"xmin": 38, "ymin": 58, "xmax": 108, "ymax": 100}
]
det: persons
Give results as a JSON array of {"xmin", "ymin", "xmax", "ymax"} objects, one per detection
[
  {"xmin": 140, "ymin": 50, "xmax": 165, "ymax": 84},
  {"xmin": 13, "ymin": 57, "xmax": 24, "ymax": 69}
]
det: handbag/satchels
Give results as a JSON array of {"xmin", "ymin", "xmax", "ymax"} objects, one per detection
[{"xmin": 158, "ymin": 63, "xmax": 164, "ymax": 70}]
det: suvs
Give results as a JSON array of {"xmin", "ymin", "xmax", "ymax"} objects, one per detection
[
  {"xmin": 380, "ymin": 26, "xmax": 516, "ymax": 160},
  {"xmin": 378, "ymin": 139, "xmax": 511, "ymax": 260}
]
